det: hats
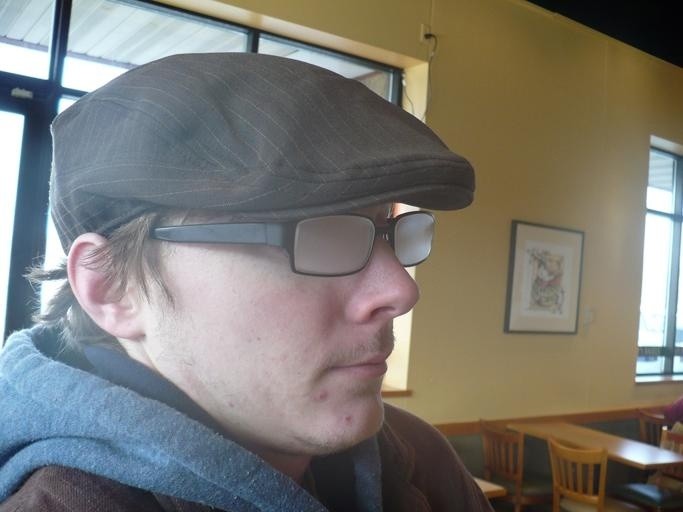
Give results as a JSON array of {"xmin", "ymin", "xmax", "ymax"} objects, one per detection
[{"xmin": 48, "ymin": 53, "xmax": 476, "ymax": 257}]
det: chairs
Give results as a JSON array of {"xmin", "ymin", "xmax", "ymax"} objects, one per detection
[{"xmin": 478, "ymin": 409, "xmax": 683, "ymax": 512}]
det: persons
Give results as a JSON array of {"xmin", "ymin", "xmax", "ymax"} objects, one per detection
[{"xmin": 0, "ymin": 53, "xmax": 497, "ymax": 512}]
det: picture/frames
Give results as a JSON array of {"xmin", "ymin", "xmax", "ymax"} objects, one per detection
[{"xmin": 503, "ymin": 220, "xmax": 586, "ymax": 336}]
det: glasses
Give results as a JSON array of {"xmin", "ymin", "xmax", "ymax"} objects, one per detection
[{"xmin": 147, "ymin": 211, "xmax": 435, "ymax": 277}]
